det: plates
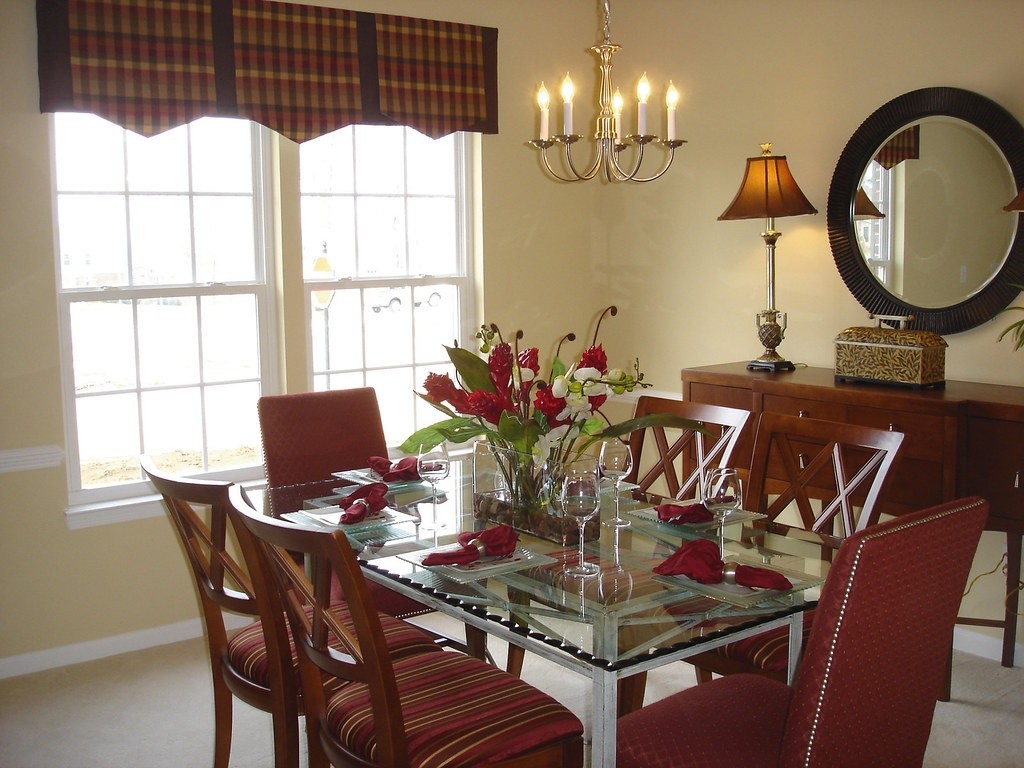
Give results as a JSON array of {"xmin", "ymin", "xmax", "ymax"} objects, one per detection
[
  {"xmin": 279, "ymin": 501, "xmax": 419, "ymax": 533},
  {"xmin": 331, "ymin": 465, "xmax": 428, "ymax": 492},
  {"xmin": 396, "ymin": 541, "xmax": 559, "ymax": 585},
  {"xmin": 623, "ymin": 499, "xmax": 768, "ymax": 529},
  {"xmin": 652, "ymin": 554, "xmax": 826, "ymax": 609},
  {"xmin": 597, "ymin": 475, "xmax": 641, "ymax": 495}
]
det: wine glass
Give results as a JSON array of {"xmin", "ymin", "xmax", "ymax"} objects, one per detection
[
  {"xmin": 598, "ymin": 440, "xmax": 634, "ymax": 528},
  {"xmin": 417, "ymin": 441, "xmax": 450, "ymax": 531},
  {"xmin": 701, "ymin": 468, "xmax": 742, "ymax": 565},
  {"xmin": 561, "ymin": 471, "xmax": 601, "ymax": 577}
]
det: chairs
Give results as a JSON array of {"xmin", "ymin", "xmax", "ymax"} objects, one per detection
[
  {"xmin": 507, "ymin": 396, "xmax": 752, "ymax": 676},
  {"xmin": 614, "ymin": 496, "xmax": 987, "ymax": 768},
  {"xmin": 141, "ymin": 455, "xmax": 444, "ymax": 768},
  {"xmin": 229, "ymin": 484, "xmax": 585, "ymax": 768},
  {"xmin": 620, "ymin": 413, "xmax": 904, "ymax": 718},
  {"xmin": 257, "ymin": 387, "xmax": 489, "ymax": 659}
]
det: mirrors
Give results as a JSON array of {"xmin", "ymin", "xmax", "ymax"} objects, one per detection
[{"xmin": 826, "ymin": 87, "xmax": 1024, "ymax": 337}]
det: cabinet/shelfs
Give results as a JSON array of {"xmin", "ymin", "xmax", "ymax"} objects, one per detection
[{"xmin": 679, "ymin": 362, "xmax": 1024, "ymax": 669}]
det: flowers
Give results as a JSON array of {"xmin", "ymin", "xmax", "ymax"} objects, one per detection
[{"xmin": 397, "ymin": 305, "xmax": 721, "ymax": 505}]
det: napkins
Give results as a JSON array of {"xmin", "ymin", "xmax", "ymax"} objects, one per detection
[
  {"xmin": 368, "ymin": 456, "xmax": 443, "ymax": 482},
  {"xmin": 338, "ymin": 482, "xmax": 390, "ymax": 523},
  {"xmin": 653, "ymin": 538, "xmax": 793, "ymax": 591},
  {"xmin": 654, "ymin": 496, "xmax": 733, "ymax": 526},
  {"xmin": 421, "ymin": 525, "xmax": 520, "ymax": 567}
]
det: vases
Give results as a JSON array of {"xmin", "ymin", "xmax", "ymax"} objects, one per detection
[{"xmin": 473, "ymin": 440, "xmax": 601, "ymax": 545}]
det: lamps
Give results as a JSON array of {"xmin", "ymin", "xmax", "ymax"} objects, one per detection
[
  {"xmin": 716, "ymin": 143, "xmax": 819, "ymax": 370},
  {"xmin": 529, "ymin": 0, "xmax": 689, "ymax": 184}
]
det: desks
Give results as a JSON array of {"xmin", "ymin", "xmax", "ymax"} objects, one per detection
[{"xmin": 260, "ymin": 453, "xmax": 845, "ymax": 768}]
